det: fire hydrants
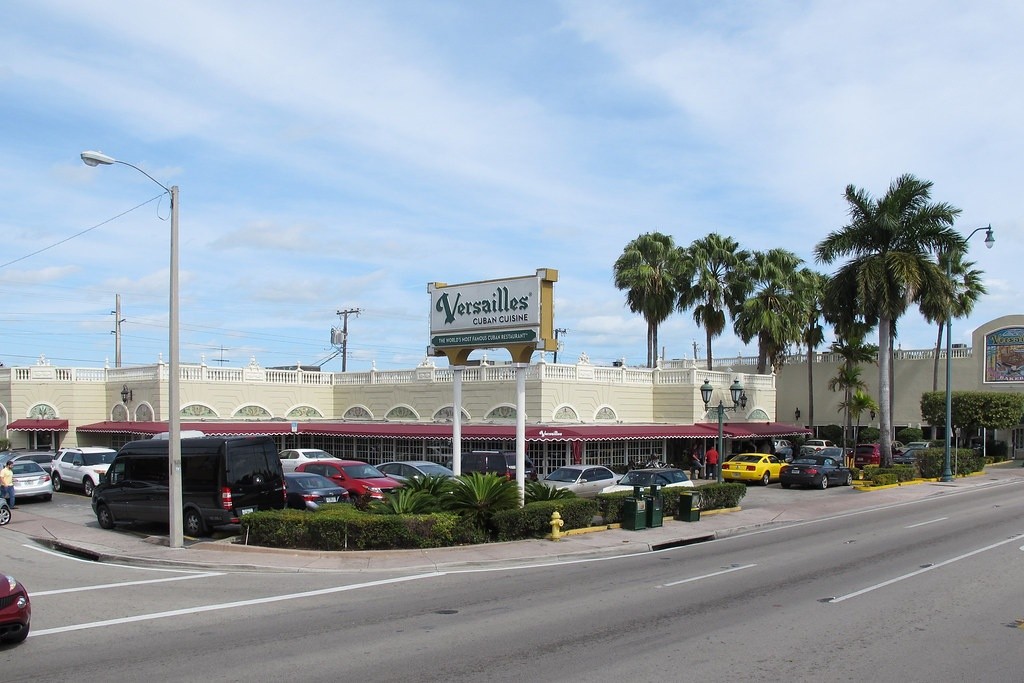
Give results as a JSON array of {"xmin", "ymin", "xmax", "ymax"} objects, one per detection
[{"xmin": 549, "ymin": 511, "xmax": 564, "ymax": 542}]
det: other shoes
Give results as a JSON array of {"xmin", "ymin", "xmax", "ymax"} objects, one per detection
[
  {"xmin": 706, "ymin": 477, "xmax": 709, "ymax": 479},
  {"xmin": 712, "ymin": 478, "xmax": 716, "ymax": 479},
  {"xmin": 10, "ymin": 507, "xmax": 18, "ymax": 509}
]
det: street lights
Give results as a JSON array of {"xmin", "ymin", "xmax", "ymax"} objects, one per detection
[
  {"xmin": 80, "ymin": 150, "xmax": 185, "ymax": 547},
  {"xmin": 940, "ymin": 223, "xmax": 997, "ymax": 482},
  {"xmin": 700, "ymin": 377, "xmax": 744, "ymax": 485}
]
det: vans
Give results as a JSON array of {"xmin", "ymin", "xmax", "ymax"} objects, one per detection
[{"xmin": 91, "ymin": 435, "xmax": 288, "ymax": 537}]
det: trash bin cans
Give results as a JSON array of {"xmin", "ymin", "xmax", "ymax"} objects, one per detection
[
  {"xmin": 680, "ymin": 491, "xmax": 700, "ymax": 522},
  {"xmin": 622, "ymin": 486, "xmax": 648, "ymax": 530},
  {"xmin": 644, "ymin": 484, "xmax": 664, "ymax": 528}
]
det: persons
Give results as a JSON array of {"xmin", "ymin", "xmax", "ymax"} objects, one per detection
[
  {"xmin": 705, "ymin": 447, "xmax": 719, "ymax": 480},
  {"xmin": 792, "ymin": 443, "xmax": 801, "ymax": 460},
  {"xmin": 689, "ymin": 444, "xmax": 704, "ymax": 479},
  {"xmin": 746, "ymin": 441, "xmax": 756, "ymax": 453},
  {"xmin": 760, "ymin": 440, "xmax": 776, "ymax": 454},
  {"xmin": 0, "ymin": 461, "xmax": 19, "ymax": 509}
]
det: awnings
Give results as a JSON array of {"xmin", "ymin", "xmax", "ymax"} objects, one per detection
[
  {"xmin": 695, "ymin": 423, "xmax": 813, "ymax": 439},
  {"xmin": 7, "ymin": 419, "xmax": 69, "ymax": 431},
  {"xmin": 76, "ymin": 421, "xmax": 728, "ymax": 442}
]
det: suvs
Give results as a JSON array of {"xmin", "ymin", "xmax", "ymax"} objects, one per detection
[
  {"xmin": 444, "ymin": 450, "xmax": 539, "ymax": 485},
  {"xmin": 51, "ymin": 446, "xmax": 124, "ymax": 497}
]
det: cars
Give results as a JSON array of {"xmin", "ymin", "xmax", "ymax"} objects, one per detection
[
  {"xmin": 0, "ymin": 572, "xmax": 32, "ymax": 645},
  {"xmin": 278, "ymin": 448, "xmax": 345, "ymax": 473},
  {"xmin": 0, "ymin": 448, "xmax": 58, "ymax": 525},
  {"xmin": 597, "ymin": 467, "xmax": 694, "ymax": 495},
  {"xmin": 294, "ymin": 460, "xmax": 404, "ymax": 505},
  {"xmin": 721, "ymin": 452, "xmax": 790, "ymax": 486},
  {"xmin": 774, "ymin": 439, "xmax": 939, "ymax": 469},
  {"xmin": 284, "ymin": 472, "xmax": 350, "ymax": 512},
  {"xmin": 364, "ymin": 461, "xmax": 454, "ymax": 494},
  {"xmin": 539, "ymin": 463, "xmax": 630, "ymax": 499},
  {"xmin": 778, "ymin": 454, "xmax": 853, "ymax": 490}
]
